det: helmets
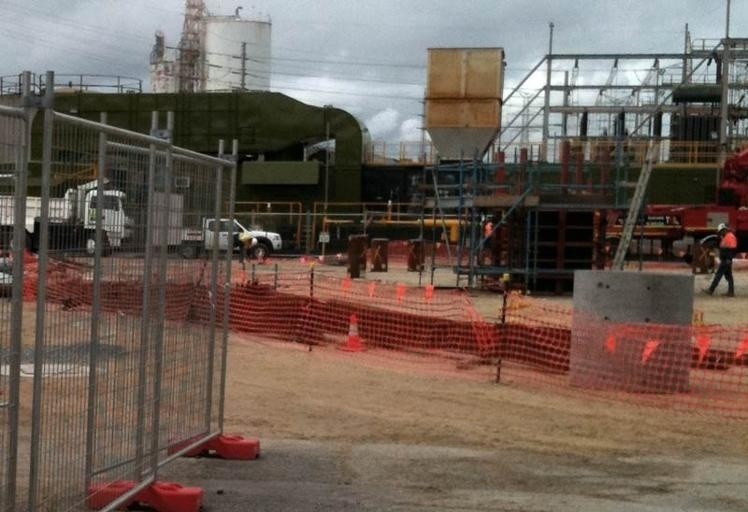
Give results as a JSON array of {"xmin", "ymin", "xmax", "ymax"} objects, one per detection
[
  {"xmin": 717, "ymin": 222, "xmax": 728, "ymax": 233},
  {"xmin": 250, "ymin": 238, "xmax": 258, "ymax": 248}
]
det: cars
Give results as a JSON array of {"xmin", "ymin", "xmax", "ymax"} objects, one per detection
[{"xmin": 0, "ymin": 243, "xmax": 12, "ymax": 297}]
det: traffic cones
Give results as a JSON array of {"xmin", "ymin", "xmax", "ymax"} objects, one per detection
[{"xmin": 337, "ymin": 314, "xmax": 369, "ymax": 355}]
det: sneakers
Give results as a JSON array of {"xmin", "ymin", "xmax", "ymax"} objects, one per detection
[
  {"xmin": 720, "ymin": 292, "xmax": 734, "ymax": 297},
  {"xmin": 702, "ymin": 289, "xmax": 713, "ymax": 296}
]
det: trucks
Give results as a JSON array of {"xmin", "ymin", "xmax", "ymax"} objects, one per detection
[
  {"xmin": 0, "ymin": 171, "xmax": 129, "ymax": 255},
  {"xmin": 602, "ymin": 148, "xmax": 747, "ymax": 264}
]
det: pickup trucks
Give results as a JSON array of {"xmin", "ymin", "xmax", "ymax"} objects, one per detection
[{"xmin": 178, "ymin": 214, "xmax": 284, "ymax": 263}]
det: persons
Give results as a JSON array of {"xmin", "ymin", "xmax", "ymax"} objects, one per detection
[
  {"xmin": 700, "ymin": 224, "xmax": 738, "ymax": 297},
  {"xmin": 233, "ymin": 231, "xmax": 258, "ymax": 271}
]
